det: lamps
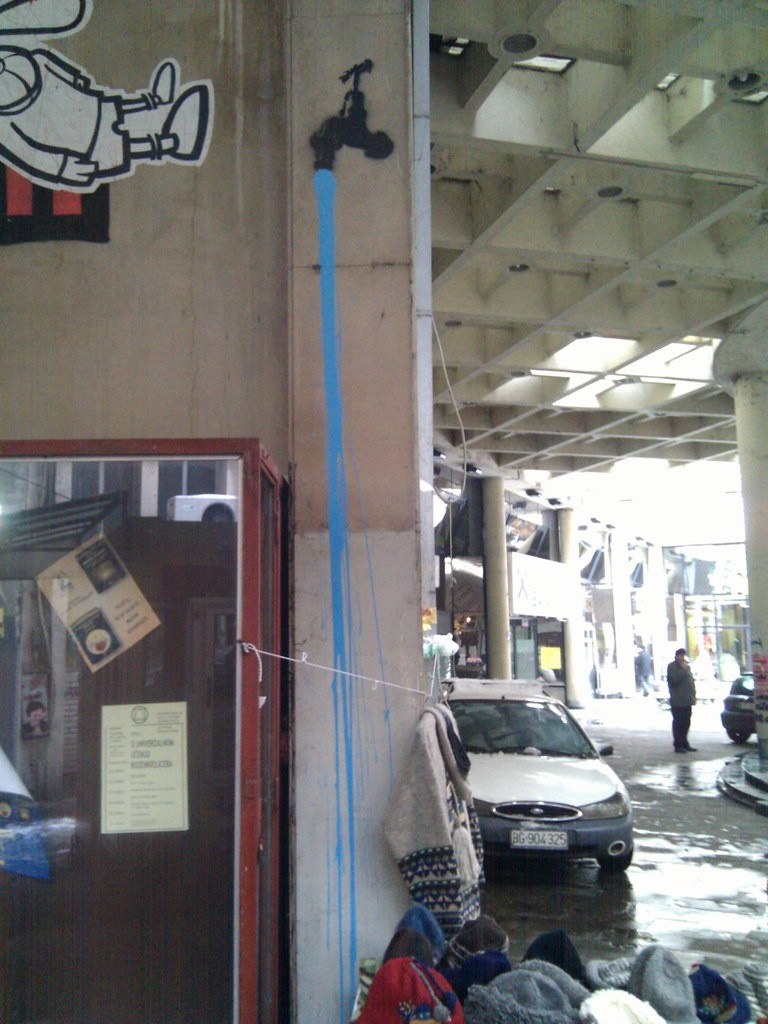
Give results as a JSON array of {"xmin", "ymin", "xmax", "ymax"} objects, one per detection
[
  {"xmin": 547, "ymin": 498, "xmax": 562, "ymax": 505},
  {"xmin": 526, "ymin": 488, "xmax": 541, "ymax": 497},
  {"xmin": 512, "ymin": 502, "xmax": 525, "ymax": 510},
  {"xmin": 462, "ymin": 464, "xmax": 482, "ymax": 474},
  {"xmin": 433, "ymin": 449, "xmax": 447, "ymax": 459}
]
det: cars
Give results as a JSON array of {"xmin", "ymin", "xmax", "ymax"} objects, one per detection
[
  {"xmin": 720, "ymin": 671, "xmax": 755, "ymax": 745},
  {"xmin": 439, "ymin": 677, "xmax": 635, "ymax": 874}
]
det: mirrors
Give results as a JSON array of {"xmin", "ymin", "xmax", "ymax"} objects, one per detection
[{"xmin": 2, "ymin": 453, "xmax": 241, "ymax": 1024}]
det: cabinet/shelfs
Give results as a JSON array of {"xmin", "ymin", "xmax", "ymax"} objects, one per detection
[{"xmin": 1, "ymin": 436, "xmax": 293, "ymax": 1024}]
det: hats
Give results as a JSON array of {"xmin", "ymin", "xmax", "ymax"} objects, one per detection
[{"xmin": 357, "ymin": 905, "xmax": 768, "ymax": 1024}]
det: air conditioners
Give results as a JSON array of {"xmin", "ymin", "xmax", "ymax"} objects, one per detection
[{"xmin": 165, "ymin": 493, "xmax": 237, "ymax": 522}]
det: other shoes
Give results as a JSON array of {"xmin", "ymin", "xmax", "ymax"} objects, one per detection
[
  {"xmin": 675, "ymin": 747, "xmax": 686, "ymax": 753},
  {"xmin": 686, "ymin": 746, "xmax": 697, "ymax": 751},
  {"xmin": 644, "ymin": 692, "xmax": 649, "ymax": 696}
]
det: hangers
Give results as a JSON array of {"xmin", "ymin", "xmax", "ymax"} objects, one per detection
[{"xmin": 424, "ymin": 694, "xmax": 437, "ymax": 708}]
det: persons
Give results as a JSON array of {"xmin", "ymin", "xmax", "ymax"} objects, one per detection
[
  {"xmin": 634, "ymin": 645, "xmax": 651, "ymax": 697},
  {"xmin": 667, "ymin": 648, "xmax": 698, "ymax": 753}
]
